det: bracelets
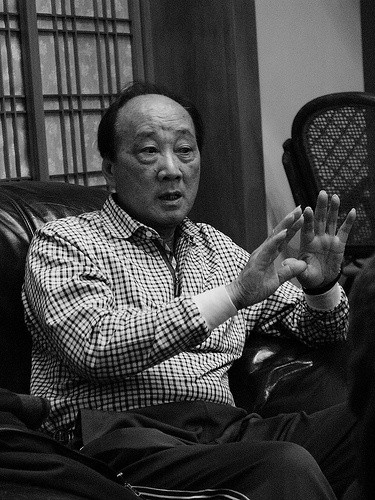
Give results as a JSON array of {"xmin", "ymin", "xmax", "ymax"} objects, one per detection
[{"xmin": 297, "ymin": 266, "xmax": 343, "ymax": 295}]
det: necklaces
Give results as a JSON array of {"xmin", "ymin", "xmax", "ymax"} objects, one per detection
[{"xmin": 159, "ymin": 231, "xmax": 177, "ymax": 245}]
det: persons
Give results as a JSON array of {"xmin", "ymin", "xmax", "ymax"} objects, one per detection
[{"xmin": 19, "ymin": 79, "xmax": 359, "ymax": 500}]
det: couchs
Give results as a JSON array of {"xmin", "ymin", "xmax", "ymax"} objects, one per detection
[{"xmin": 0, "ymin": 179, "xmax": 352, "ymax": 499}]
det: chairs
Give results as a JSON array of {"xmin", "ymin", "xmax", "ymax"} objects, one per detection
[{"xmin": 282, "ymin": 92, "xmax": 374, "ymax": 277}]
